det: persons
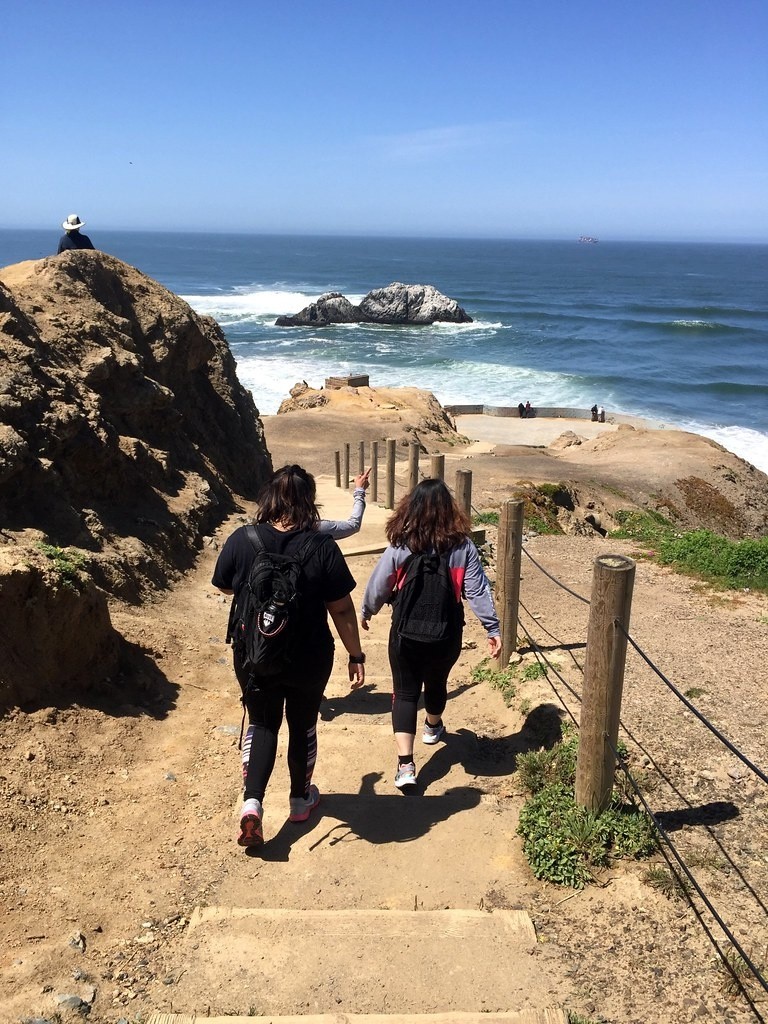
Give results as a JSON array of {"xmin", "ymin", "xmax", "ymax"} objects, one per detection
[
  {"xmin": 525, "ymin": 401, "xmax": 530, "ymax": 418},
  {"xmin": 56, "ymin": 214, "xmax": 95, "ymax": 255},
  {"xmin": 212, "ymin": 465, "xmax": 365, "ymax": 847},
  {"xmin": 361, "ymin": 479, "xmax": 503, "ymax": 788},
  {"xmin": 590, "ymin": 404, "xmax": 598, "ymax": 421},
  {"xmin": 518, "ymin": 403, "xmax": 524, "ymax": 419},
  {"xmin": 597, "ymin": 406, "xmax": 604, "ymax": 422},
  {"xmin": 316, "ymin": 466, "xmax": 373, "ymax": 539}
]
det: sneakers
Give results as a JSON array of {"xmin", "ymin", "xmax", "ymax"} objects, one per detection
[
  {"xmin": 289, "ymin": 784, "xmax": 320, "ymax": 821},
  {"xmin": 395, "ymin": 762, "xmax": 417, "ymax": 787},
  {"xmin": 236, "ymin": 798, "xmax": 264, "ymax": 846},
  {"xmin": 422, "ymin": 722, "xmax": 444, "ymax": 743}
]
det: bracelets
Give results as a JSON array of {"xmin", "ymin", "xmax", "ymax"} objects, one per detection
[{"xmin": 349, "ymin": 652, "xmax": 366, "ymax": 664}]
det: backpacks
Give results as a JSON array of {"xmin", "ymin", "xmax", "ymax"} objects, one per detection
[
  {"xmin": 226, "ymin": 524, "xmax": 333, "ymax": 700},
  {"xmin": 386, "ymin": 536, "xmax": 465, "ymax": 671}
]
